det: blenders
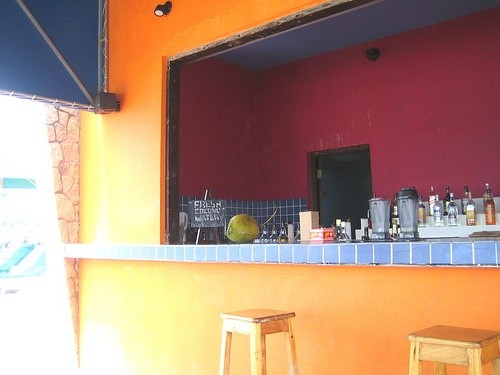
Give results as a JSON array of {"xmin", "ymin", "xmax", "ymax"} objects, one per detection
[
  {"xmin": 368, "ymin": 197, "xmax": 392, "ymax": 242},
  {"xmin": 396, "ymin": 188, "xmax": 422, "ymax": 242}
]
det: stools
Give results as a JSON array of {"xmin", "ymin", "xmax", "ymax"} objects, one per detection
[
  {"xmin": 409, "ymin": 326, "xmax": 500, "ymax": 375},
  {"xmin": 218, "ymin": 309, "xmax": 298, "ymax": 375}
]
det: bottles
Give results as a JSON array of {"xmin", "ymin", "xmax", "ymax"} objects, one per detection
[
  {"xmin": 443, "ymin": 185, "xmax": 451, "ymax": 215},
  {"xmin": 482, "ymin": 183, "xmax": 493, "ymax": 214},
  {"xmin": 418, "ymin": 201, "xmax": 426, "ymax": 225},
  {"xmin": 392, "ymin": 194, "xmax": 400, "ymax": 228},
  {"xmin": 448, "ymin": 192, "xmax": 459, "ymax": 226},
  {"xmin": 433, "ymin": 195, "xmax": 442, "ymax": 222},
  {"xmin": 465, "ymin": 191, "xmax": 477, "ymax": 226},
  {"xmin": 428, "ymin": 185, "xmax": 436, "ymax": 216},
  {"xmin": 461, "ymin": 186, "xmax": 469, "ymax": 215},
  {"xmin": 484, "ymin": 189, "xmax": 496, "ymax": 225}
]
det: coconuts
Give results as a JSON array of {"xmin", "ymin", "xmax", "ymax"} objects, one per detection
[{"xmin": 226, "ymin": 214, "xmax": 262, "ymax": 243}]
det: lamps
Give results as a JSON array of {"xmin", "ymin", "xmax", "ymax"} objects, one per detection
[{"xmin": 154, "ymin": 1, "xmax": 172, "ymax": 17}]
